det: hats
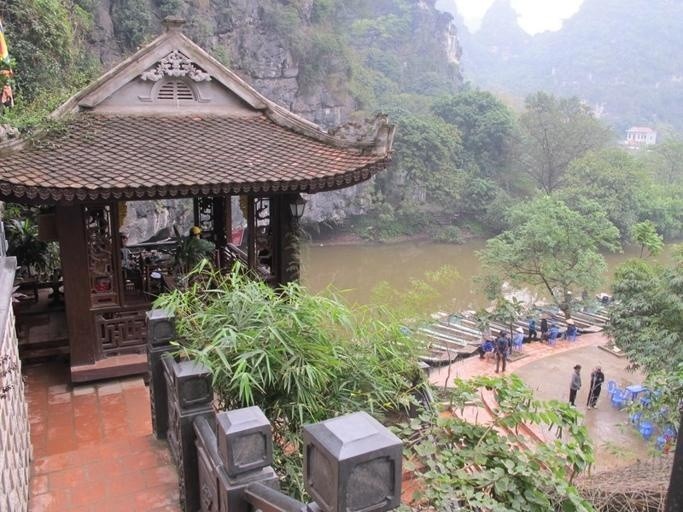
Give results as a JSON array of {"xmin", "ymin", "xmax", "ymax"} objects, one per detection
[{"xmin": 565, "ymin": 318, "xmax": 575, "ymax": 325}]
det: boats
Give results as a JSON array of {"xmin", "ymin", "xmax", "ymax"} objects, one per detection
[{"xmin": 388, "ymin": 301, "xmax": 617, "ymax": 377}]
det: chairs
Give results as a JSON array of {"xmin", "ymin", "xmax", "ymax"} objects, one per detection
[
  {"xmin": 513, "ymin": 337, "xmax": 523, "ymax": 348},
  {"xmin": 568, "ymin": 327, "xmax": 578, "ymax": 341},
  {"xmin": 120, "ymin": 248, "xmax": 220, "ymax": 303},
  {"xmin": 608, "ymin": 380, "xmax": 677, "ymax": 450},
  {"xmin": 549, "ymin": 330, "xmax": 560, "ymax": 343}
]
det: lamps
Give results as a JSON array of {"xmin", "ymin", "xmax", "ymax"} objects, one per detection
[{"xmin": 290, "ymin": 192, "xmax": 307, "ymax": 224}]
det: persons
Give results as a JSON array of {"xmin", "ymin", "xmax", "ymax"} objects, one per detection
[
  {"xmin": 184, "ymin": 225, "xmax": 218, "ymax": 276},
  {"xmin": 120, "ymin": 230, "xmax": 145, "ymax": 292},
  {"xmin": 569, "ymin": 364, "xmax": 582, "ymax": 407},
  {"xmin": 495, "ymin": 331, "xmax": 508, "ymax": 374},
  {"xmin": 587, "ymin": 366, "xmax": 605, "ymax": 409},
  {"xmin": 477, "ymin": 317, "xmax": 576, "ymax": 360}
]
